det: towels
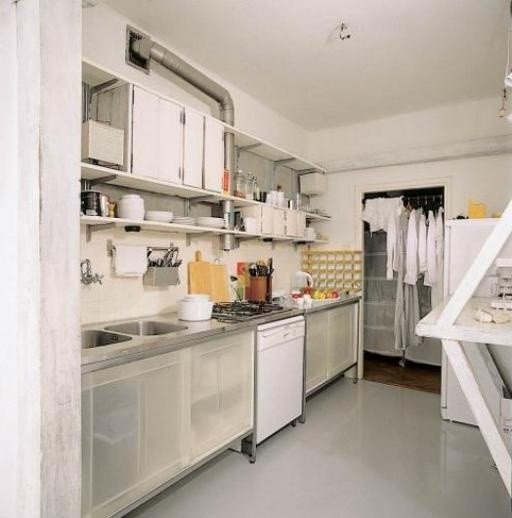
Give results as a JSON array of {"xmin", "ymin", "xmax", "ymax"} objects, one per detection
[{"xmin": 111, "ymin": 243, "xmax": 148, "ymax": 278}]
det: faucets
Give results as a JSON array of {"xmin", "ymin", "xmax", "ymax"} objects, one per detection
[{"xmin": 81, "ymin": 258, "xmax": 103, "ymax": 285}]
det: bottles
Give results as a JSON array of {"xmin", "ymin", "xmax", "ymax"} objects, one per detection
[
  {"xmin": 234, "ymin": 205, "xmax": 241, "ymax": 232},
  {"xmin": 233, "ymin": 169, "xmax": 260, "ymax": 204},
  {"xmin": 117, "ymin": 195, "xmax": 145, "ymax": 220}
]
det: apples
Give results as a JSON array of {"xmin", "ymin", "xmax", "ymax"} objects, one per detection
[{"xmin": 312, "ymin": 288, "xmax": 338, "ymax": 299}]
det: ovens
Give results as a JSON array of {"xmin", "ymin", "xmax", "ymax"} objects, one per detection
[{"xmin": 256, "ymin": 315, "xmax": 304, "ymax": 446}]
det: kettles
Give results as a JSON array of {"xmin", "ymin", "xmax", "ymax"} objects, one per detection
[
  {"xmin": 291, "ymin": 271, "xmax": 313, "ymax": 295},
  {"xmin": 229, "ymin": 275, "xmax": 243, "ymax": 302}
]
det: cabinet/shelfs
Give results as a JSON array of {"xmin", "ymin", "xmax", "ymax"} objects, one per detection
[
  {"xmin": 82, "ymin": 58, "xmax": 330, "ymax": 245},
  {"xmin": 304, "ymin": 302, "xmax": 357, "ymax": 393},
  {"xmin": 80, "ymin": 331, "xmax": 254, "ymax": 518}
]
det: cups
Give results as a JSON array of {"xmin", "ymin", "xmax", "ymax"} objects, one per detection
[{"xmin": 260, "ymin": 189, "xmax": 284, "ymax": 208}]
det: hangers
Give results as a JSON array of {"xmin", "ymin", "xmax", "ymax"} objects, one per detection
[{"xmin": 388, "ymin": 195, "xmax": 444, "ymax": 225}]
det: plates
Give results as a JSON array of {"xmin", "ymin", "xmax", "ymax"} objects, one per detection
[
  {"xmin": 171, "ymin": 216, "xmax": 196, "ymax": 226},
  {"xmin": 182, "ymin": 294, "xmax": 210, "ymax": 302}
]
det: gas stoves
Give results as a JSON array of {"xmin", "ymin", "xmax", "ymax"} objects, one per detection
[{"xmin": 211, "ymin": 299, "xmax": 293, "ymax": 323}]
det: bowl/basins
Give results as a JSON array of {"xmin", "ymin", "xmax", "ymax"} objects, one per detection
[
  {"xmin": 177, "ymin": 299, "xmax": 213, "ymax": 321},
  {"xmin": 146, "ymin": 211, "xmax": 173, "ymax": 222},
  {"xmin": 196, "ymin": 217, "xmax": 224, "ymax": 229}
]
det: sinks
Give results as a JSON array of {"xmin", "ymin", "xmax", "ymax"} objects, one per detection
[
  {"xmin": 104, "ymin": 320, "xmax": 189, "ymax": 336},
  {"xmin": 82, "ymin": 330, "xmax": 132, "ymax": 351}
]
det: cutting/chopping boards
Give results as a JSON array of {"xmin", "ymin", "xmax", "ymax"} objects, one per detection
[{"xmin": 187, "ymin": 262, "xmax": 232, "ymax": 305}]
